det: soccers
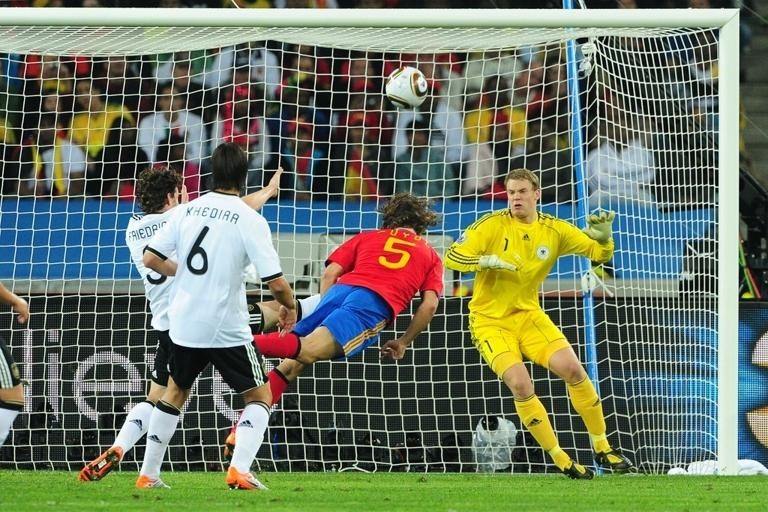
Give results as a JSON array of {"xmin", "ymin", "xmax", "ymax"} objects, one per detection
[{"xmin": 385, "ymin": 66, "xmax": 428, "ymax": 108}]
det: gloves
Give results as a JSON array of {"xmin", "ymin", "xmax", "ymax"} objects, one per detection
[
  {"xmin": 479, "ymin": 254, "xmax": 524, "ymax": 272},
  {"xmin": 582, "ymin": 210, "xmax": 615, "ymax": 244}
]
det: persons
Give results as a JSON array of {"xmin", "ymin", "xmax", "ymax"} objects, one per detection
[
  {"xmin": 1, "ymin": 1, "xmax": 754, "ymax": 206},
  {"xmin": 444, "ymin": 168, "xmax": 634, "ymax": 479},
  {"xmin": 224, "ymin": 193, "xmax": 444, "ymax": 463},
  {"xmin": 78, "ymin": 166, "xmax": 320, "ymax": 481},
  {"xmin": 0, "ymin": 282, "xmax": 28, "ymax": 448},
  {"xmin": 135, "ymin": 142, "xmax": 298, "ymax": 489}
]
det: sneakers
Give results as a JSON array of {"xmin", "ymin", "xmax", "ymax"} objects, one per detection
[
  {"xmin": 137, "ymin": 474, "xmax": 170, "ymax": 489},
  {"xmin": 594, "ymin": 447, "xmax": 633, "ymax": 468},
  {"xmin": 225, "ymin": 430, "xmax": 235, "ymax": 461},
  {"xmin": 226, "ymin": 467, "xmax": 269, "ymax": 490},
  {"xmin": 79, "ymin": 446, "xmax": 122, "ymax": 481},
  {"xmin": 564, "ymin": 458, "xmax": 594, "ymax": 479}
]
